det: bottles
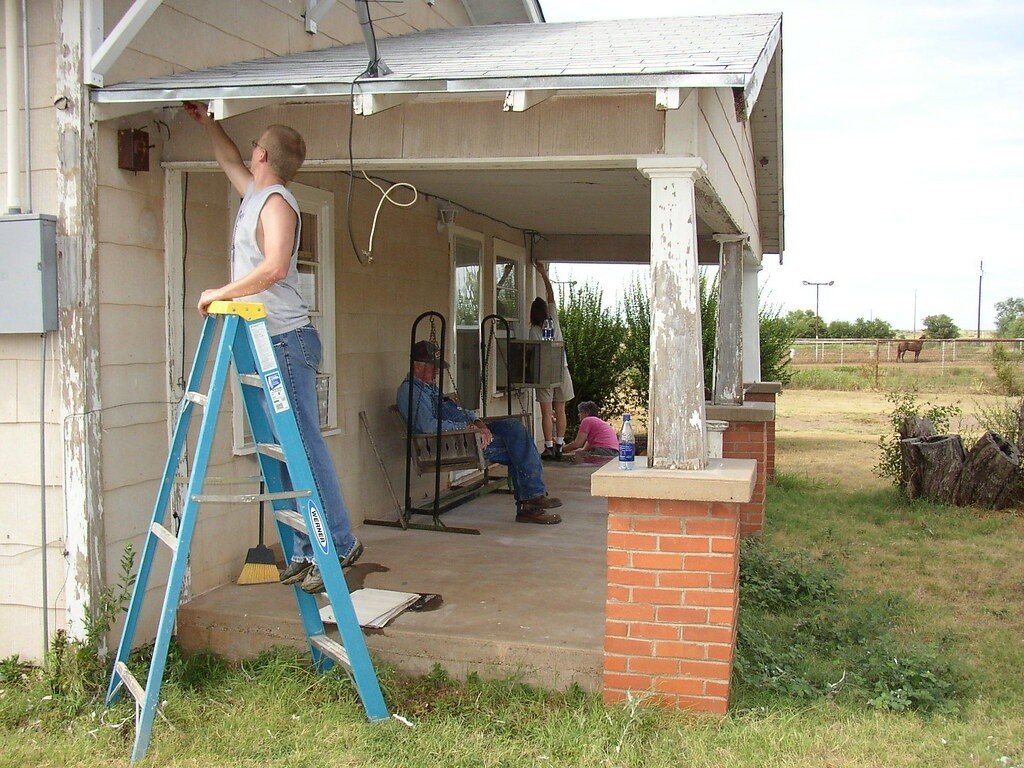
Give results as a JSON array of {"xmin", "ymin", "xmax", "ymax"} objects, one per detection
[
  {"xmin": 549, "ymin": 318, "xmax": 554, "ymax": 341},
  {"xmin": 619, "ymin": 416, "xmax": 635, "ymax": 470},
  {"xmin": 542, "ymin": 318, "xmax": 549, "ymax": 340}
]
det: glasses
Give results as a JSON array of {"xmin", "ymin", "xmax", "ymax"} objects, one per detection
[{"xmin": 252, "ymin": 139, "xmax": 267, "ymax": 163}]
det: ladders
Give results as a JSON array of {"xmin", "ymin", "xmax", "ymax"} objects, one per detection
[{"xmin": 99, "ymin": 297, "xmax": 392, "ymax": 762}]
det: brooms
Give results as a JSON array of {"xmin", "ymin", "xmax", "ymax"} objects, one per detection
[{"xmin": 236, "ymin": 470, "xmax": 280, "ymax": 585}]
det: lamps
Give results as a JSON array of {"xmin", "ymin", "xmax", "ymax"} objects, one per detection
[{"xmin": 437, "ymin": 199, "xmax": 459, "ymax": 233}]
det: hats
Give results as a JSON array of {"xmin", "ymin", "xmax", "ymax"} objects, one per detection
[{"xmin": 410, "ymin": 340, "xmax": 451, "ymax": 368}]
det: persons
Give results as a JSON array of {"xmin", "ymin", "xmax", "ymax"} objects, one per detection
[
  {"xmin": 183, "ymin": 98, "xmax": 364, "ymax": 593},
  {"xmin": 528, "ymin": 261, "xmax": 619, "ymax": 456},
  {"xmin": 396, "ymin": 340, "xmax": 563, "ymax": 526}
]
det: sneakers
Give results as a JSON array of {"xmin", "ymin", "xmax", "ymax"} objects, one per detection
[
  {"xmin": 515, "ymin": 501, "xmax": 561, "ymax": 524},
  {"xmin": 280, "ymin": 559, "xmax": 314, "ymax": 585},
  {"xmin": 300, "ymin": 537, "xmax": 364, "ymax": 594},
  {"xmin": 535, "ymin": 496, "xmax": 561, "ymax": 508},
  {"xmin": 541, "ymin": 443, "xmax": 564, "ymax": 461}
]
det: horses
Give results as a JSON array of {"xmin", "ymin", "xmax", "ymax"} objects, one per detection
[{"xmin": 895, "ymin": 335, "xmax": 926, "ymax": 362}]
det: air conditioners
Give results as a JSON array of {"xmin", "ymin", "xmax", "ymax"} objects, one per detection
[{"xmin": 508, "ymin": 339, "xmax": 566, "ymax": 390}]
